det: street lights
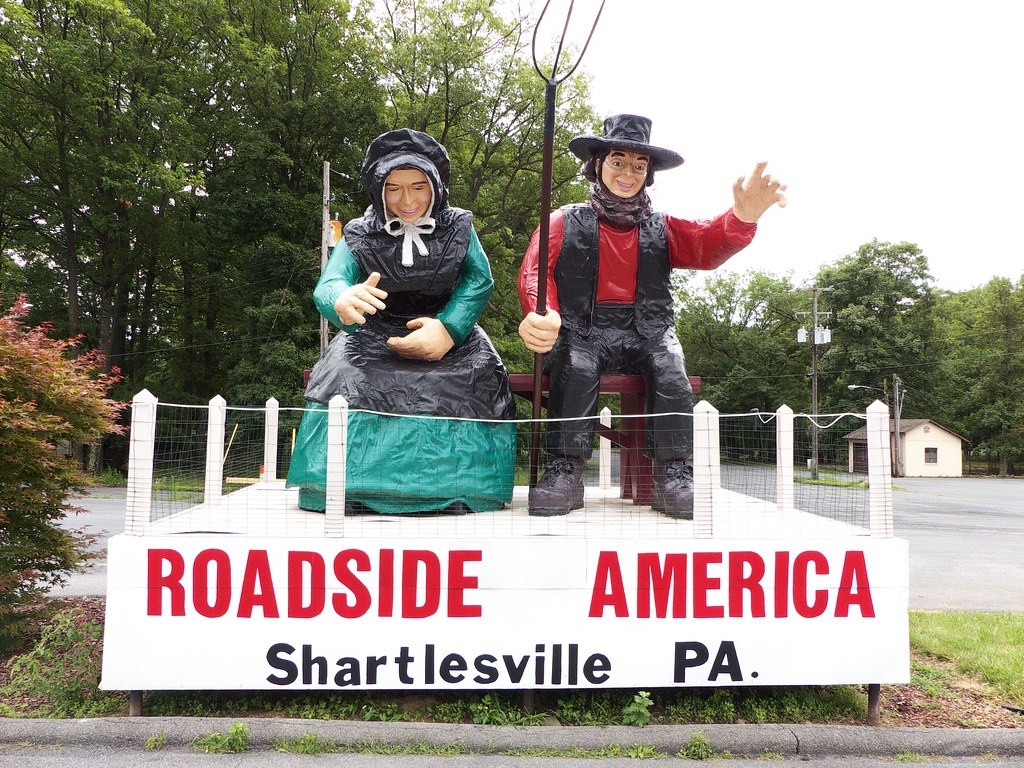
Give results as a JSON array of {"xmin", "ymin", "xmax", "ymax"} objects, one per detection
[
  {"xmin": 848, "ymin": 385, "xmax": 889, "ymax": 406},
  {"xmin": 797, "ymin": 325, "xmax": 832, "ymax": 480}
]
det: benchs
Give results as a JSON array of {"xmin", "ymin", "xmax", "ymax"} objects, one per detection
[{"xmin": 302, "ymin": 370, "xmax": 700, "ymax": 506}]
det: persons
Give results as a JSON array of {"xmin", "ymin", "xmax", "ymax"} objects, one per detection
[
  {"xmin": 518, "ymin": 114, "xmax": 787, "ymax": 519},
  {"xmin": 285, "ymin": 127, "xmax": 516, "ymax": 516}
]
